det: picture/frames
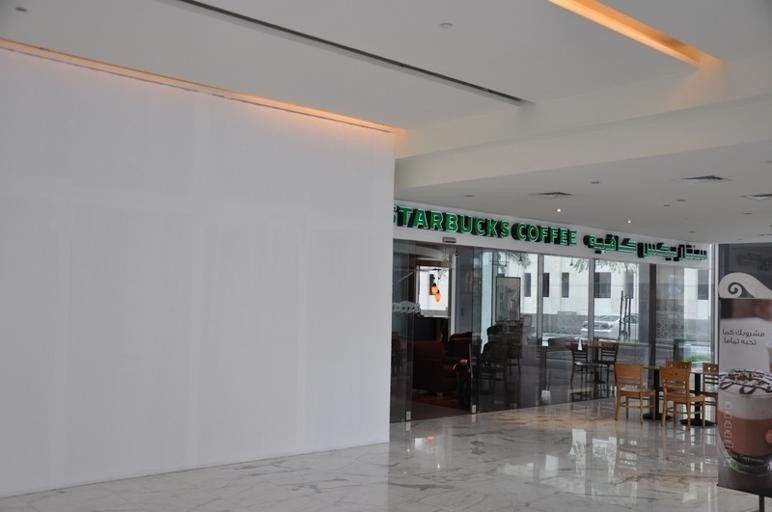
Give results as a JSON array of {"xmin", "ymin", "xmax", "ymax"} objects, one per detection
[{"xmin": 496, "ymin": 275, "xmax": 520, "ymax": 322}]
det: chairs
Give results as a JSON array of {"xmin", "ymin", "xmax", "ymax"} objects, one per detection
[
  {"xmin": 613, "ymin": 358, "xmax": 717, "ymax": 429},
  {"xmin": 568, "ymin": 340, "xmax": 618, "ymax": 392},
  {"xmin": 408, "ymin": 341, "xmax": 464, "ymax": 393}
]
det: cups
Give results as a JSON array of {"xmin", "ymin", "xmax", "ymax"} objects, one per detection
[{"xmin": 716, "ymin": 372, "xmax": 772, "ymax": 475}]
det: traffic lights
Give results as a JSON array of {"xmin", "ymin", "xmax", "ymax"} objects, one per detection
[{"xmin": 429, "ymin": 273, "xmax": 435, "ymax": 295}]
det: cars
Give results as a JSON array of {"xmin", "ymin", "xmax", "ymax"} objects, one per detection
[{"xmin": 581, "ymin": 312, "xmax": 639, "ymax": 343}]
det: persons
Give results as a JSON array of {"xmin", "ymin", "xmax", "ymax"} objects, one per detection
[{"xmin": 483, "ymin": 326, "xmax": 504, "ymax": 368}]
